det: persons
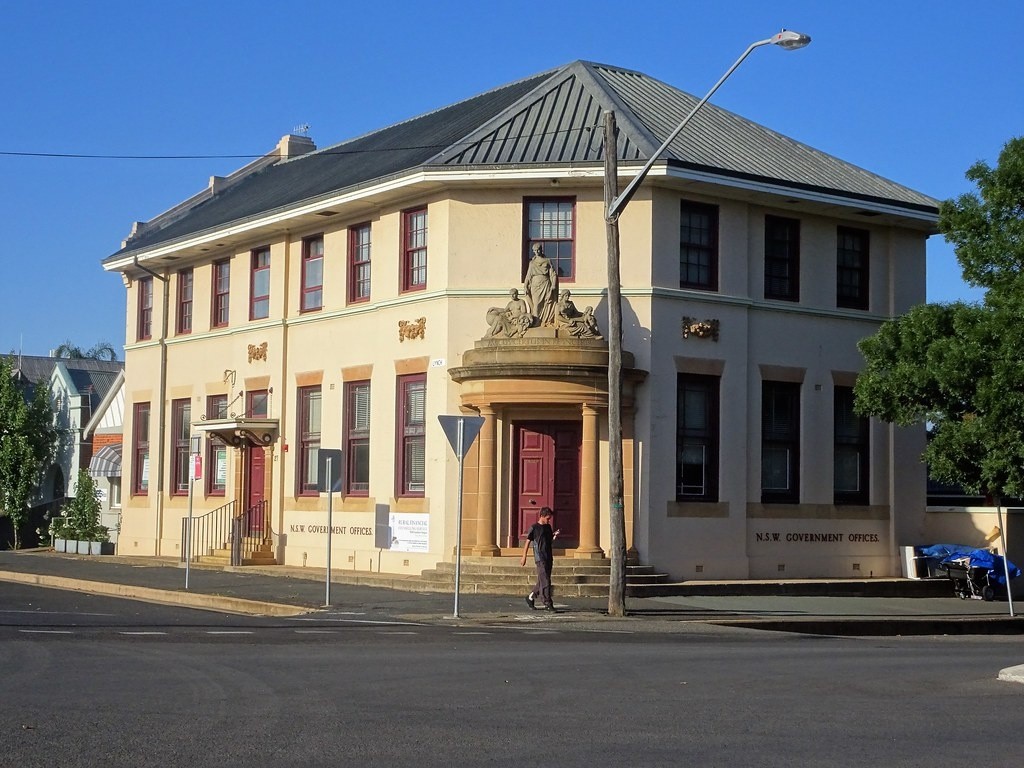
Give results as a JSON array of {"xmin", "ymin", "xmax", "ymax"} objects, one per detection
[
  {"xmin": 488, "ymin": 242, "xmax": 603, "ymax": 338},
  {"xmin": 520, "ymin": 507, "xmax": 560, "ymax": 612}
]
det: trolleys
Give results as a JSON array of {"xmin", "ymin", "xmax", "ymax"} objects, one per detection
[{"xmin": 940, "ymin": 560, "xmax": 1000, "ymax": 601}]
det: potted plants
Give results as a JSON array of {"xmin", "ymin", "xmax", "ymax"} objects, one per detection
[
  {"xmin": 71, "ymin": 468, "xmax": 102, "ymax": 555},
  {"xmin": 91, "ymin": 525, "xmax": 113, "ymax": 555},
  {"xmin": 59, "ymin": 526, "xmax": 78, "ymax": 554}
]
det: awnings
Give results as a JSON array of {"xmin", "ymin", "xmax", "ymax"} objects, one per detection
[{"xmin": 88, "ymin": 443, "xmax": 122, "ymax": 477}]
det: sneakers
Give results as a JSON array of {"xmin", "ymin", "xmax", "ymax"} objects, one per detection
[
  {"xmin": 523, "ymin": 595, "xmax": 537, "ymax": 611},
  {"xmin": 543, "ymin": 606, "xmax": 556, "ymax": 613}
]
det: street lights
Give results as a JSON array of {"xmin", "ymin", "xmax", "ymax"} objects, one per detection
[{"xmin": 604, "ymin": 30, "xmax": 813, "ymax": 618}]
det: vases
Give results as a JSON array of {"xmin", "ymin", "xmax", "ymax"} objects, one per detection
[{"xmin": 55, "ymin": 538, "xmax": 67, "ymax": 553}]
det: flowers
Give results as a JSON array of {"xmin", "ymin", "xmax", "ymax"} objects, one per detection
[{"xmin": 36, "ymin": 510, "xmax": 68, "ymax": 539}]
